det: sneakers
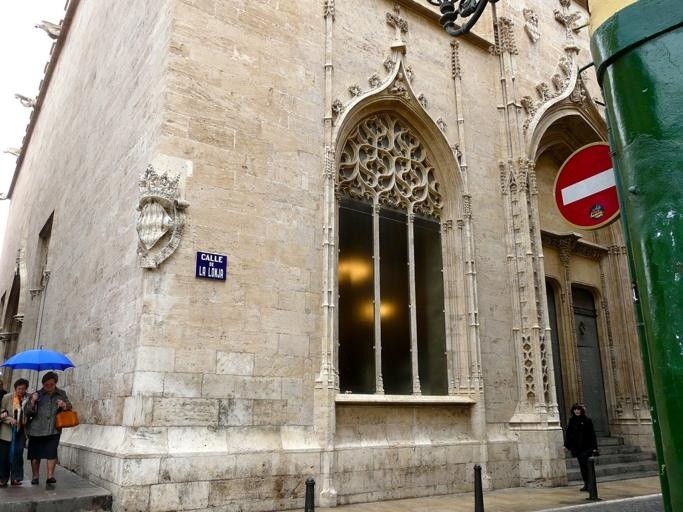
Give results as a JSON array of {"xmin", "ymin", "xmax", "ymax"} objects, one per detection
[
  {"xmin": 579, "ymin": 486, "xmax": 587, "ymax": 491},
  {"xmin": 0, "ymin": 480, "xmax": 22, "ymax": 488}
]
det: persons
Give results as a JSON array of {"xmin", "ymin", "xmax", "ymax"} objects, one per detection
[
  {"xmin": 23, "ymin": 371, "xmax": 71, "ymax": 483},
  {"xmin": 563, "ymin": 402, "xmax": 600, "ymax": 499},
  {"xmin": 0, "ymin": 378, "xmax": 32, "ymax": 485},
  {"xmin": 0, "ymin": 379, "xmax": 7, "ymax": 401}
]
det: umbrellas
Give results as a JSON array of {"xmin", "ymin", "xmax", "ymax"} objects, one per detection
[{"xmin": 0, "ymin": 347, "xmax": 75, "ymax": 394}]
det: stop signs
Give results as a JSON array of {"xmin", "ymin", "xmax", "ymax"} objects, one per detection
[{"xmin": 553, "ymin": 142, "xmax": 624, "ymax": 234}]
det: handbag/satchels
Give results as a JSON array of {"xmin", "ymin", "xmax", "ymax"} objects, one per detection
[{"xmin": 55, "ymin": 410, "xmax": 79, "ymax": 429}]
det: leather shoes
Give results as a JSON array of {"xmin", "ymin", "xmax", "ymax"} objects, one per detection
[
  {"xmin": 46, "ymin": 477, "xmax": 56, "ymax": 483},
  {"xmin": 32, "ymin": 479, "xmax": 39, "ymax": 484}
]
